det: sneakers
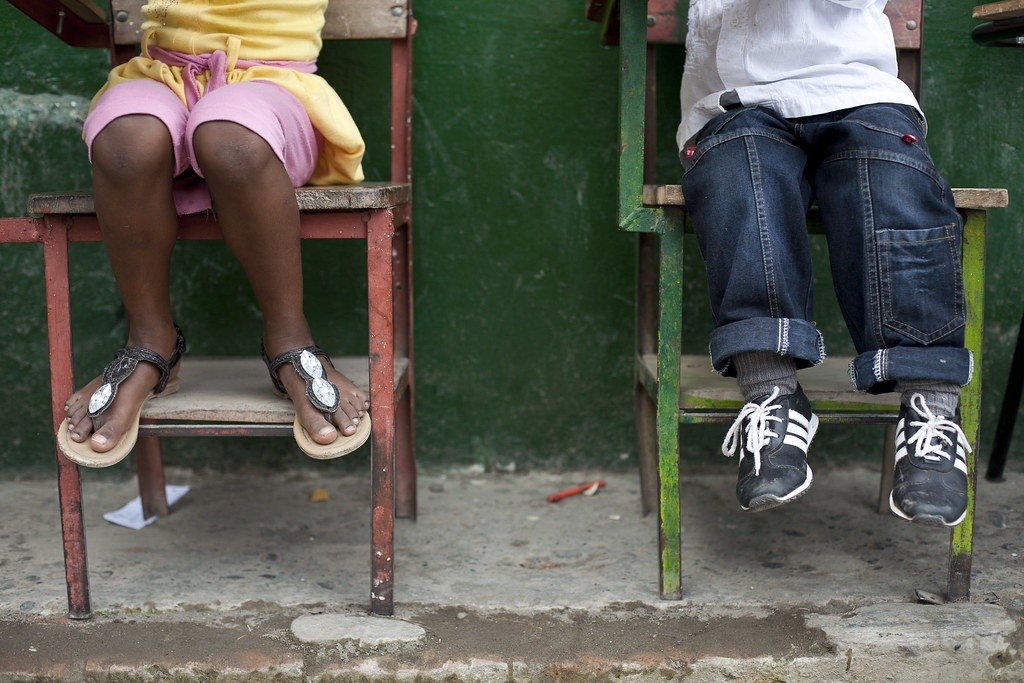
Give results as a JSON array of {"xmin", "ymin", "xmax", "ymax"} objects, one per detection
[
  {"xmin": 722, "ymin": 378, "xmax": 821, "ymax": 512},
  {"xmin": 890, "ymin": 392, "xmax": 973, "ymax": 527}
]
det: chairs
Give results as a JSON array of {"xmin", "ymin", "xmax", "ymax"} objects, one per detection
[
  {"xmin": 586, "ymin": 0, "xmax": 1009, "ymax": 600},
  {"xmin": 0, "ymin": 0, "xmax": 416, "ymax": 619}
]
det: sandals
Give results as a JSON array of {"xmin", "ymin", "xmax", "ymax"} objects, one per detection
[
  {"xmin": 57, "ymin": 323, "xmax": 186, "ymax": 467},
  {"xmin": 261, "ymin": 330, "xmax": 372, "ymax": 461}
]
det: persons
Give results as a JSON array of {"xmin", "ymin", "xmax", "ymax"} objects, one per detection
[
  {"xmin": 675, "ymin": 0, "xmax": 974, "ymax": 527},
  {"xmin": 57, "ymin": 1, "xmax": 373, "ymax": 469}
]
